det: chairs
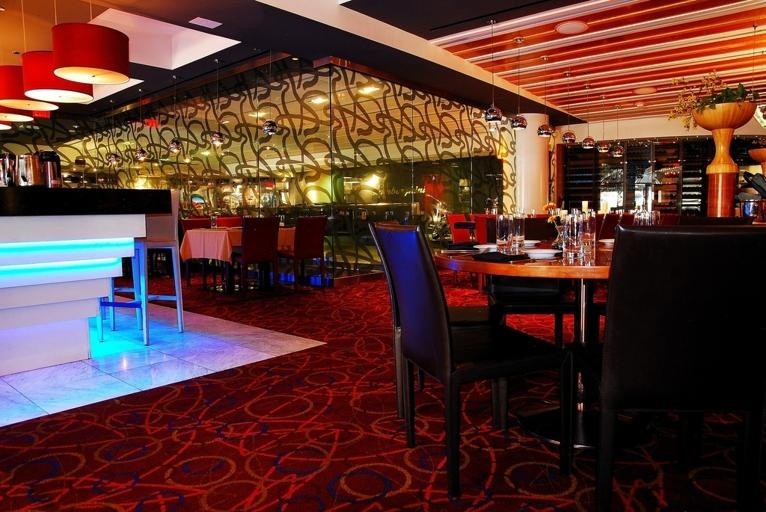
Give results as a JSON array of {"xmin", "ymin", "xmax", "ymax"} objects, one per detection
[
  {"xmin": 568, "ymin": 220, "xmax": 765, "ymax": 511},
  {"xmin": 447, "ymin": 213, "xmax": 497, "ymax": 247},
  {"xmin": 370, "ymin": 223, "xmax": 567, "ymax": 498},
  {"xmin": 370, "ymin": 221, "xmax": 492, "ymax": 420},
  {"xmin": 523, "ymin": 217, "xmax": 559, "ymax": 240},
  {"xmin": 122, "ymin": 216, "xmax": 327, "ymax": 294},
  {"xmin": 595, "ymin": 212, "xmax": 636, "ymax": 241},
  {"xmin": 98, "ymin": 190, "xmax": 184, "ymax": 345}
]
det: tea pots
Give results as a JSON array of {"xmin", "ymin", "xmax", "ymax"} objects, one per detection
[{"xmin": 1, "ymin": 150, "xmax": 61, "ymax": 188}]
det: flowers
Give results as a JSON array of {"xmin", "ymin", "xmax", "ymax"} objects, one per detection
[
  {"xmin": 665, "ymin": 71, "xmax": 759, "ymax": 130},
  {"xmin": 548, "ymin": 214, "xmax": 556, "ymax": 223}
]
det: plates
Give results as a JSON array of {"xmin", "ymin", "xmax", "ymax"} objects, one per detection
[
  {"xmin": 522, "ymin": 249, "xmax": 563, "ymax": 259},
  {"xmin": 524, "ymin": 239, "xmax": 541, "ymax": 247},
  {"xmin": 473, "ymin": 244, "xmax": 498, "ymax": 253},
  {"xmin": 599, "ymin": 237, "xmax": 614, "ymax": 249}
]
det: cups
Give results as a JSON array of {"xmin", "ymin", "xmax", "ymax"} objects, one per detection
[
  {"xmin": 563, "ymin": 213, "xmax": 594, "ymax": 250},
  {"xmin": 496, "ymin": 214, "xmax": 525, "ymax": 247},
  {"xmin": 740, "ymin": 199, "xmax": 766, "ymax": 222}
]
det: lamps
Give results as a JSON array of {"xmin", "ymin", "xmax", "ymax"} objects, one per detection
[
  {"xmin": 52, "ymin": 0, "xmax": 129, "ymax": 84},
  {"xmin": 0, "ymin": 107, "xmax": 34, "ymax": 133},
  {"xmin": 485, "ymin": 19, "xmax": 624, "ymax": 158},
  {"xmin": 0, "ymin": 0, "xmax": 59, "ymax": 112},
  {"xmin": 20, "ymin": 1, "xmax": 94, "ymax": 104}
]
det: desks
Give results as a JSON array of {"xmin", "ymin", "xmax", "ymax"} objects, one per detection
[{"xmin": 432, "ymin": 245, "xmax": 616, "ymax": 451}]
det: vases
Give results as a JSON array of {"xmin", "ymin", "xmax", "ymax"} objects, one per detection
[{"xmin": 692, "ymin": 101, "xmax": 758, "ymax": 173}]
